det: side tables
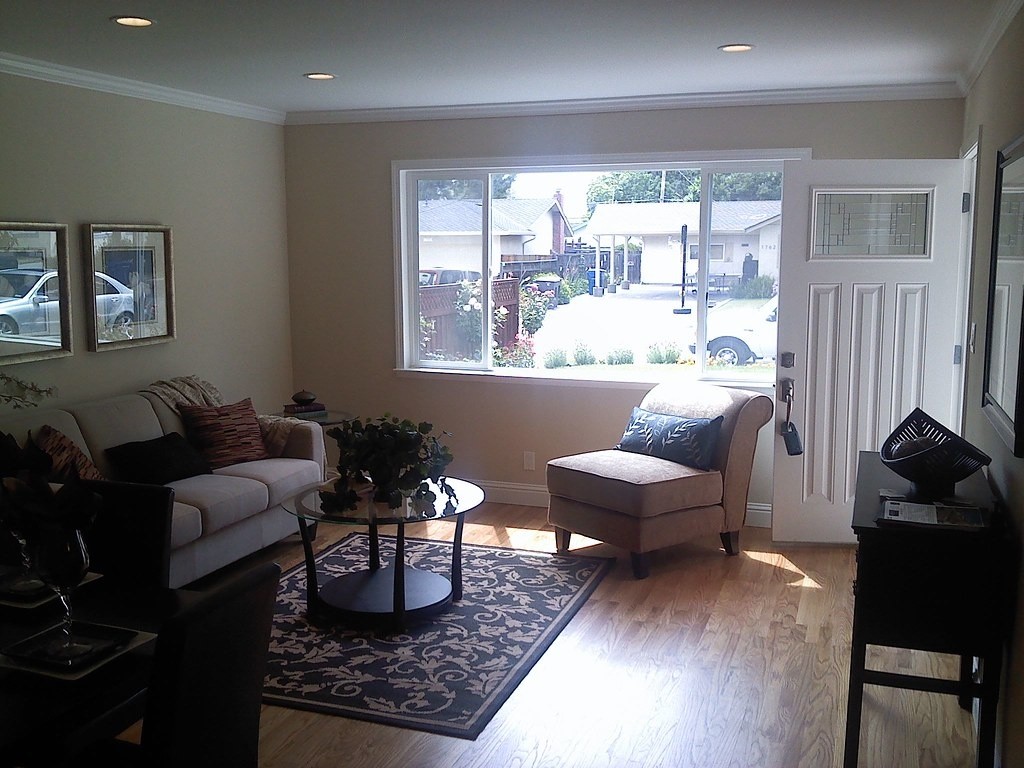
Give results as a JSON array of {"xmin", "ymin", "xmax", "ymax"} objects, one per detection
[{"xmin": 271, "ymin": 413, "xmax": 360, "ymax": 424}]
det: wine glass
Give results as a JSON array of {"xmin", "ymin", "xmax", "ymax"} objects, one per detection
[{"xmin": 35, "ymin": 528, "xmax": 91, "ymax": 659}]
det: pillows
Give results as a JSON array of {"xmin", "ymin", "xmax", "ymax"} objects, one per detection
[
  {"xmin": 174, "ymin": 396, "xmax": 274, "ymax": 470},
  {"xmin": 614, "ymin": 405, "xmax": 723, "ymax": 472},
  {"xmin": 103, "ymin": 432, "xmax": 214, "ymax": 486},
  {"xmin": 35, "ymin": 425, "xmax": 109, "ymax": 480}
]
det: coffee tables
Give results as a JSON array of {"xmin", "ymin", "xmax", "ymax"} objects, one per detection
[{"xmin": 280, "ymin": 476, "xmax": 486, "ymax": 630}]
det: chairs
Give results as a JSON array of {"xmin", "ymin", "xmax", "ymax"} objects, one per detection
[
  {"xmin": 545, "ymin": 379, "xmax": 774, "ymax": 581},
  {"xmin": 64, "ymin": 481, "xmax": 281, "ymax": 768}
]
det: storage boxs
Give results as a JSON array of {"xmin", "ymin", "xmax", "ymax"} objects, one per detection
[{"xmin": 0, "ymin": 570, "xmax": 104, "ymax": 626}]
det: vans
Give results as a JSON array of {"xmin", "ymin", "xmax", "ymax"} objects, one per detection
[
  {"xmin": 462, "ymin": 271, "xmax": 482, "ymax": 283},
  {"xmin": 418, "ymin": 268, "xmax": 463, "ymax": 287}
]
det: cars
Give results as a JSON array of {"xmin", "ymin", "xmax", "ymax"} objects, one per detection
[
  {"xmin": 0, "ymin": 268, "xmax": 146, "ymax": 340},
  {"xmin": 689, "ymin": 295, "xmax": 779, "ymax": 365}
]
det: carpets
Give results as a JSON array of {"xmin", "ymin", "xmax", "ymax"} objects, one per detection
[{"xmin": 259, "ymin": 531, "xmax": 617, "ymax": 742}]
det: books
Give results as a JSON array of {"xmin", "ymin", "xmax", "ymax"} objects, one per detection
[
  {"xmin": 283, "ymin": 404, "xmax": 328, "ymax": 419},
  {"xmin": 318, "ymin": 477, "xmax": 373, "ymax": 497},
  {"xmin": 874, "ymin": 501, "xmax": 990, "ymax": 532}
]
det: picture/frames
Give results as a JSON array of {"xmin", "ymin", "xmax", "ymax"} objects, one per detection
[
  {"xmin": 0, "ymin": 221, "xmax": 74, "ymax": 366},
  {"xmin": 83, "ymin": 222, "xmax": 177, "ymax": 354}
]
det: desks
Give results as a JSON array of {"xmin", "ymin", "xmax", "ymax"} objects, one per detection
[
  {"xmin": 270, "ymin": 412, "xmax": 360, "ymax": 426},
  {"xmin": 843, "ymin": 451, "xmax": 1016, "ymax": 768}
]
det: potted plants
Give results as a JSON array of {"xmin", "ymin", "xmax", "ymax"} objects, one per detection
[{"xmin": 313, "ymin": 412, "xmax": 459, "ymax": 521}]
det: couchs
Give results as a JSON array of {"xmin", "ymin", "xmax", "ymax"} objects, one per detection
[{"xmin": 0, "ymin": 375, "xmax": 325, "ymax": 590}]
